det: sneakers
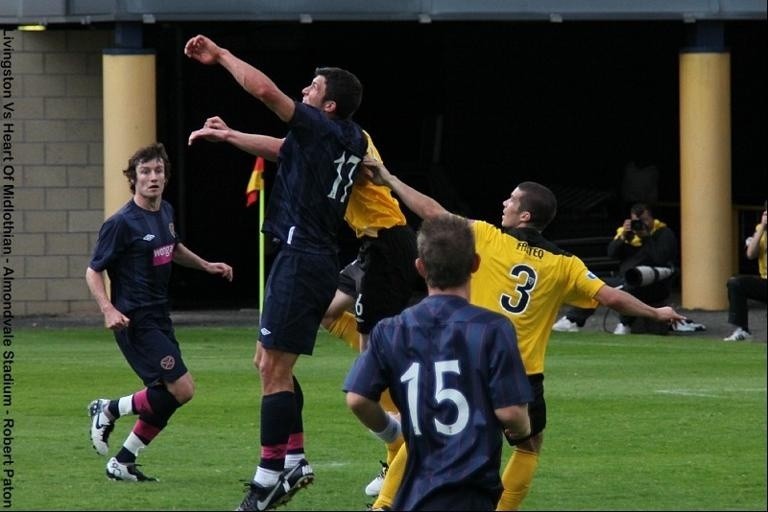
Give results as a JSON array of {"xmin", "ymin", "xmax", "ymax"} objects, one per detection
[
  {"xmin": 613, "ymin": 322, "xmax": 632, "ymax": 335},
  {"xmin": 552, "ymin": 316, "xmax": 579, "ymax": 332},
  {"xmin": 106, "ymin": 456, "xmax": 159, "ymax": 482},
  {"xmin": 88, "ymin": 399, "xmax": 114, "ymax": 456},
  {"xmin": 236, "ymin": 458, "xmax": 314, "ymax": 511},
  {"xmin": 365, "ymin": 460, "xmax": 389, "ymax": 496},
  {"xmin": 724, "ymin": 328, "xmax": 753, "ymax": 340}
]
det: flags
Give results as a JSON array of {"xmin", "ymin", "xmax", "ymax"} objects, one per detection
[{"xmin": 244, "ymin": 152, "xmax": 264, "ymax": 206}]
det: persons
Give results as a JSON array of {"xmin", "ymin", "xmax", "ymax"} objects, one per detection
[
  {"xmin": 182, "ymin": 34, "xmax": 369, "ymax": 510},
  {"xmin": 362, "ymin": 156, "xmax": 691, "ymax": 511},
  {"xmin": 341, "ymin": 210, "xmax": 536, "ymax": 511},
  {"xmin": 83, "ymin": 140, "xmax": 235, "ymax": 485},
  {"xmin": 188, "ymin": 116, "xmax": 429, "ymax": 497},
  {"xmin": 551, "ymin": 203, "xmax": 681, "ymax": 335},
  {"xmin": 723, "ymin": 209, "xmax": 768, "ymax": 343}
]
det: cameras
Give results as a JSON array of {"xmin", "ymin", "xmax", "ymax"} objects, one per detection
[{"xmin": 631, "ymin": 217, "xmax": 647, "ymax": 232}]
